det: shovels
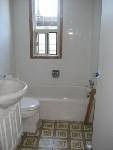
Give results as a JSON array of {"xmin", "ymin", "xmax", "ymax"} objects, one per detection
[{"xmin": 84, "ymin": 87, "xmax": 96, "ymax": 126}]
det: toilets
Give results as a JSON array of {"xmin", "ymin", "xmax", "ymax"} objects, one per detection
[{"xmin": 20, "ymin": 98, "xmax": 40, "ymax": 133}]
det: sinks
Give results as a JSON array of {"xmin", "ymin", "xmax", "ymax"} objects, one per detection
[{"xmin": 0, "ymin": 78, "xmax": 25, "ymax": 98}]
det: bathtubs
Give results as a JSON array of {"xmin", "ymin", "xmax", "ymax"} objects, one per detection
[{"xmin": 24, "ymin": 85, "xmax": 95, "ymax": 121}]
